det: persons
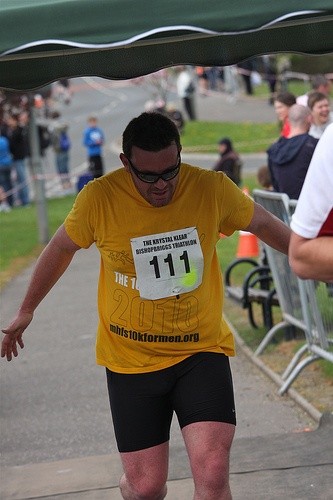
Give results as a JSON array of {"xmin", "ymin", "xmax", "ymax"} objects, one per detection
[
  {"xmin": 268, "ymin": 104, "xmax": 320, "ymax": 203},
  {"xmin": 286, "ymin": 119, "xmax": 331, "ymax": 283},
  {"xmin": 308, "ymin": 93, "xmax": 333, "ymax": 137},
  {"xmin": 177, "ymin": 66, "xmax": 200, "ymax": 120},
  {"xmin": 274, "ymin": 91, "xmax": 295, "ymax": 138},
  {"xmin": 82, "ymin": 118, "xmax": 104, "ymax": 178},
  {"xmin": 197, "ymin": 54, "xmax": 291, "ymax": 102},
  {"xmin": 145, "ymin": 98, "xmax": 184, "ymax": 133},
  {"xmin": 0, "ymin": 78, "xmax": 76, "ymax": 213},
  {"xmin": 258, "ymin": 165, "xmax": 275, "ymax": 193},
  {"xmin": 0, "ymin": 112, "xmax": 292, "ymax": 500},
  {"xmin": 209, "ymin": 136, "xmax": 241, "ymax": 185},
  {"xmin": 295, "ymin": 75, "xmax": 330, "ymax": 107}
]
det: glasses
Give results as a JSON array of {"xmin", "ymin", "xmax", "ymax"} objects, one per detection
[{"xmin": 126, "ymin": 150, "xmax": 181, "ymax": 183}]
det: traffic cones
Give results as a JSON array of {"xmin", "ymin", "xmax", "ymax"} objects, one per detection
[{"xmin": 235, "ymin": 187, "xmax": 261, "ymax": 258}]
